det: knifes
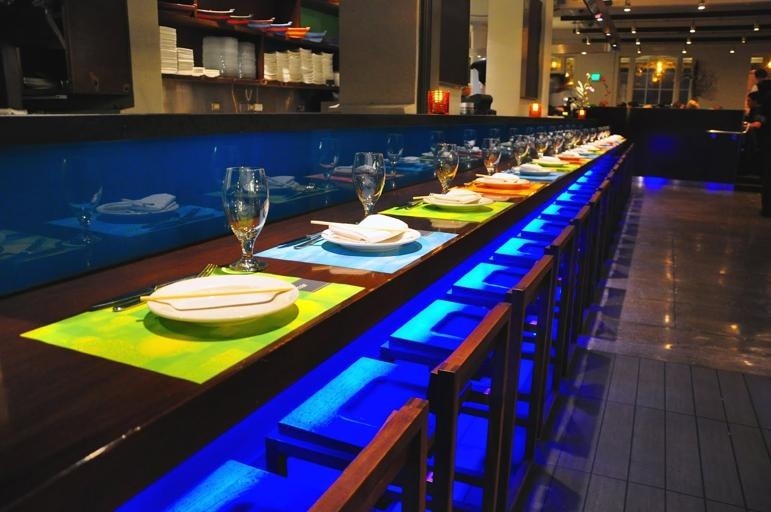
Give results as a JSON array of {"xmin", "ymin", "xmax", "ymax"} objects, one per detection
[
  {"xmin": 90, "ymin": 273, "xmax": 197, "ymax": 311},
  {"xmin": 176, "ymin": 208, "xmax": 199, "ymax": 226}
]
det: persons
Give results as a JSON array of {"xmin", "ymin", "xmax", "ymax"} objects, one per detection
[{"xmin": 744, "ymin": 67, "xmax": 771, "ymax": 218}]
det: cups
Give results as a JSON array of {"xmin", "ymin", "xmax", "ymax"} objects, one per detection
[{"xmin": 459, "ymin": 102, "xmax": 475, "ymax": 116}]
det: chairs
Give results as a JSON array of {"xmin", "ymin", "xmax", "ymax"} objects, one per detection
[
  {"xmin": 448, "ymin": 225, "xmax": 577, "ymax": 396},
  {"xmin": 264, "ymin": 302, "xmax": 512, "ymax": 512},
  {"xmin": 488, "ymin": 140, "xmax": 635, "ymax": 382},
  {"xmin": 163, "ymin": 397, "xmax": 430, "ymax": 512},
  {"xmin": 380, "ymin": 254, "xmax": 555, "ymax": 465}
]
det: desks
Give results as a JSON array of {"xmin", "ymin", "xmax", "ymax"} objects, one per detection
[{"xmin": 2, "ymin": 133, "xmax": 624, "ymax": 512}]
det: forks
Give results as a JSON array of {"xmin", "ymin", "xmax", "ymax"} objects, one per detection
[
  {"xmin": 1, "ymin": 236, "xmax": 50, "ymax": 258},
  {"xmin": 112, "ymin": 262, "xmax": 218, "ymax": 315}
]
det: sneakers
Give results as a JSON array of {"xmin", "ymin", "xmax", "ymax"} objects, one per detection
[{"xmin": 759, "ymin": 208, "xmax": 771, "ymax": 217}]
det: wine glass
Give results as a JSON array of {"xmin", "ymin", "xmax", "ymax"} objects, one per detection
[
  {"xmin": 220, "ymin": 166, "xmax": 271, "ymax": 274},
  {"xmin": 384, "ymin": 132, "xmax": 406, "ymax": 177},
  {"xmin": 317, "ymin": 136, "xmax": 341, "ymax": 190},
  {"xmin": 352, "ymin": 151, "xmax": 386, "ymax": 215},
  {"xmin": 432, "ymin": 141, "xmax": 459, "ymax": 193},
  {"xmin": 463, "ymin": 127, "xmax": 477, "ymax": 162},
  {"xmin": 428, "ymin": 129, "xmax": 447, "ymax": 155},
  {"xmin": 535, "ymin": 126, "xmax": 612, "ymax": 167},
  {"xmin": 65, "ymin": 176, "xmax": 104, "ymax": 266}
]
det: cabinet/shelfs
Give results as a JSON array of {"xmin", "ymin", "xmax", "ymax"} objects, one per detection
[{"xmin": 156, "ymin": 0, "xmax": 338, "ymax": 92}]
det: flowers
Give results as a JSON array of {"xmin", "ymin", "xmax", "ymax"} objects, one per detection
[{"xmin": 571, "ymin": 69, "xmax": 594, "ymax": 109}]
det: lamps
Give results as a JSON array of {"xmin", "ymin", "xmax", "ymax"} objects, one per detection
[{"xmin": 565, "ymin": 0, "xmax": 761, "ymax": 56}]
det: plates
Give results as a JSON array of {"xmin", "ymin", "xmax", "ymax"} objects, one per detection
[
  {"xmin": 145, "ymin": 273, "xmax": 299, "ymax": 327},
  {"xmin": 96, "ymin": 201, "xmax": 179, "ymax": 215},
  {"xmin": 172, "ymin": 1, "xmax": 328, "ymax": 42},
  {"xmin": 422, "ymin": 196, "xmax": 494, "ymax": 211},
  {"xmin": 160, "ymin": 24, "xmax": 196, "ymax": 73},
  {"xmin": 264, "ymin": 48, "xmax": 340, "ymax": 86},
  {"xmin": 402, "ymin": 157, "xmax": 417, "ymax": 166},
  {"xmin": 270, "ymin": 185, "xmax": 299, "ymax": 192},
  {"xmin": 201, "ymin": 35, "xmax": 256, "ymax": 79},
  {"xmin": 479, "ymin": 135, "xmax": 625, "ymax": 188},
  {"xmin": 335, "ymin": 169, "xmax": 352, "ymax": 176},
  {"xmin": 320, "ymin": 228, "xmax": 419, "ymax": 253}
]
